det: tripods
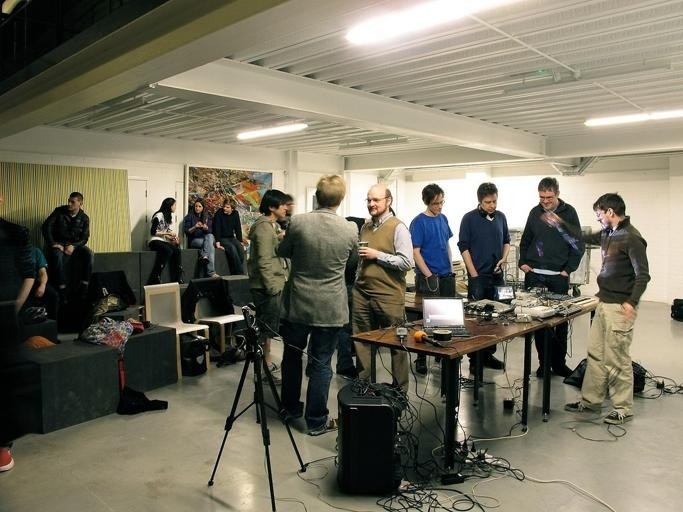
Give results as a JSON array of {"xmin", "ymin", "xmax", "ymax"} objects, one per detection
[{"xmin": 208, "ymin": 304, "xmax": 306, "ymax": 512}]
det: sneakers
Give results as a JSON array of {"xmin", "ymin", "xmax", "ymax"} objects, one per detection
[
  {"xmin": 254, "ymin": 361, "xmax": 282, "ymax": 385},
  {"xmin": 484, "ymin": 354, "xmax": 505, "ymax": 369},
  {"xmin": 415, "ymin": 354, "xmax": 427, "ymax": 374},
  {"xmin": 310, "ymin": 418, "xmax": 338, "ymax": 437},
  {"xmin": 563, "ymin": 397, "xmax": 602, "ymax": 415},
  {"xmin": 536, "ymin": 364, "xmax": 552, "ymax": 376},
  {"xmin": 552, "ymin": 360, "xmax": 572, "ymax": 376},
  {"xmin": 469, "ymin": 360, "xmax": 476, "ymax": 374},
  {"xmin": 603, "ymin": 406, "xmax": 633, "ymax": 424},
  {"xmin": 0, "ymin": 447, "xmax": 15, "ymax": 473}
]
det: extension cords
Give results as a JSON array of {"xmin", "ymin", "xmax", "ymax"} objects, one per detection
[{"xmin": 453, "ymin": 445, "xmax": 500, "ymax": 461}]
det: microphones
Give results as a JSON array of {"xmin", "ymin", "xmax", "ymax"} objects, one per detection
[{"xmin": 414, "ymin": 331, "xmax": 442, "ymax": 347}]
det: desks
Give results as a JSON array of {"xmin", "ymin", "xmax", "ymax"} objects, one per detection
[{"xmin": 348, "ymin": 288, "xmax": 602, "ymax": 471}]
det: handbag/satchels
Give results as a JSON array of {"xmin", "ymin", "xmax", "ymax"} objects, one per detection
[{"xmin": 414, "ymin": 272, "xmax": 456, "ymax": 296}]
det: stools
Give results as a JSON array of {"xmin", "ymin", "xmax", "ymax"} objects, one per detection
[{"xmin": 0, "ymin": 248, "xmax": 256, "ymax": 436}]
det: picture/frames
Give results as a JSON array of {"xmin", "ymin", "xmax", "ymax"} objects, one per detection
[{"xmin": 183, "ymin": 163, "xmax": 275, "ymax": 249}]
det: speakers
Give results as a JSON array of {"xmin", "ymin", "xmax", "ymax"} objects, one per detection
[{"xmin": 337, "ymin": 382, "xmax": 401, "ymax": 496}]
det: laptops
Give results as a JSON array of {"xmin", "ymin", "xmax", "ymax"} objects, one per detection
[{"xmin": 422, "ymin": 297, "xmax": 470, "ymax": 337}]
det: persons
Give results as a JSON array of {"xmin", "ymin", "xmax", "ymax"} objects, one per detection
[
  {"xmin": 409, "ymin": 184, "xmax": 454, "ymax": 376},
  {"xmin": 545, "ymin": 191, "xmax": 651, "ymax": 425},
  {"xmin": 458, "ymin": 182, "xmax": 512, "ymax": 376},
  {"xmin": 517, "ymin": 177, "xmax": 585, "ymax": 378}
]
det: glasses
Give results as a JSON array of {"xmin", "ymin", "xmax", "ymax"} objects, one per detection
[{"xmin": 364, "ymin": 196, "xmax": 388, "ymax": 203}]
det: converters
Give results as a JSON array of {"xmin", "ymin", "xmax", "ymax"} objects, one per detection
[
  {"xmin": 504, "ymin": 397, "xmax": 514, "ymax": 408},
  {"xmin": 396, "ymin": 326, "xmax": 408, "ymax": 336},
  {"xmin": 441, "ymin": 473, "xmax": 464, "ymax": 485}
]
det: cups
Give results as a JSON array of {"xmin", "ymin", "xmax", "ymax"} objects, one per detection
[{"xmin": 357, "ymin": 241, "xmax": 368, "ymax": 256}]
